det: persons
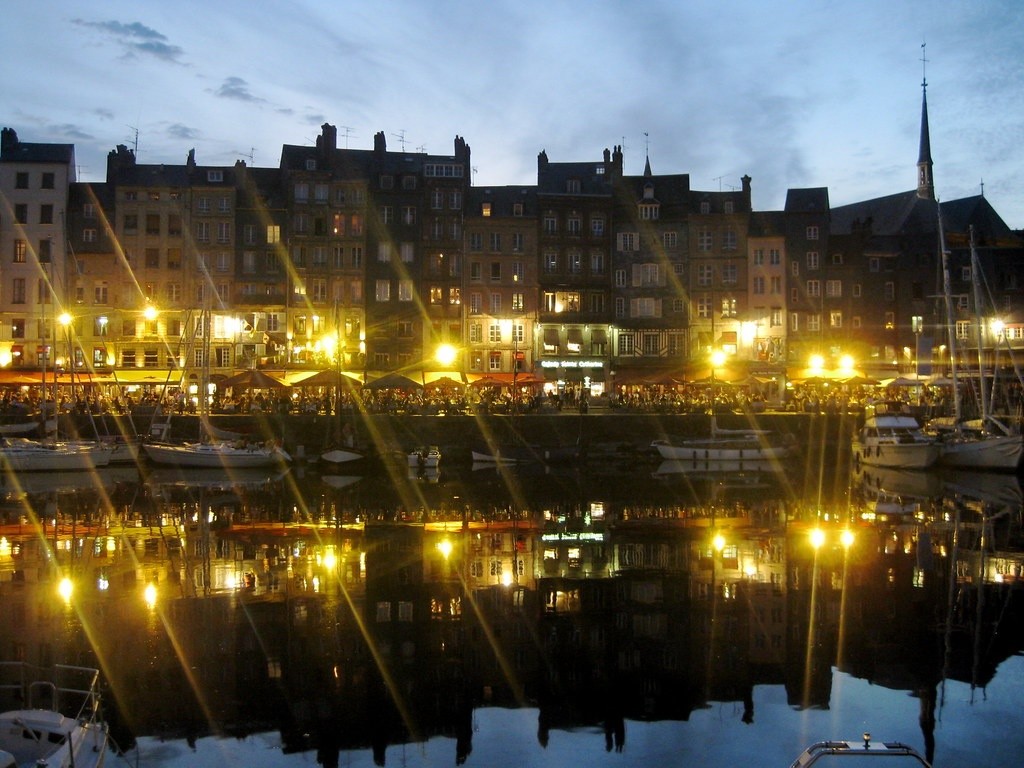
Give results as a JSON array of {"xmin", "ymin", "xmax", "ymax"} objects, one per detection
[
  {"xmin": 780, "ymin": 387, "xmax": 955, "ymax": 410},
  {"xmin": 1, "ymin": 384, "xmax": 589, "ymax": 414},
  {"xmin": 608, "ymin": 384, "xmax": 766, "ymax": 414}
]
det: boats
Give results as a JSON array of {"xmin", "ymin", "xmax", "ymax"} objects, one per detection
[
  {"xmin": 851, "ymin": 399, "xmax": 937, "ymax": 469},
  {"xmin": 0, "ymin": 661, "xmax": 109, "ymax": 768},
  {"xmin": 408, "ymin": 447, "xmax": 441, "ymax": 468}
]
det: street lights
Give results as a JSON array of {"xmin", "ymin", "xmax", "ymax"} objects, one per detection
[
  {"xmin": 512, "ymin": 336, "xmax": 518, "ymax": 397},
  {"xmin": 912, "ymin": 313, "xmax": 922, "ymax": 403}
]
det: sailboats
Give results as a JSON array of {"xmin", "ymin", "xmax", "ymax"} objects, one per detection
[
  {"xmin": 144, "ymin": 291, "xmax": 292, "ymax": 467},
  {"xmin": 0, "ymin": 333, "xmax": 143, "ymax": 471},
  {"xmin": 651, "ymin": 295, "xmax": 801, "ymax": 459},
  {"xmin": 920, "ymin": 199, "xmax": 1024, "ymax": 468},
  {"xmin": 650, "ymin": 459, "xmax": 788, "ymax": 590},
  {"xmin": 318, "ymin": 367, "xmax": 375, "ymax": 476}
]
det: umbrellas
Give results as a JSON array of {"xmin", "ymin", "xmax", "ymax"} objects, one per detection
[
  {"xmin": 888, "ymin": 377, "xmax": 922, "ymax": 395},
  {"xmin": 611, "ymin": 375, "xmax": 647, "ymax": 394},
  {"xmin": 742, "ymin": 375, "xmax": 774, "ymax": 385},
  {"xmin": 841, "ymin": 376, "xmax": 881, "ymax": 390},
  {"xmin": 929, "ymin": 377, "xmax": 964, "ymax": 387},
  {"xmin": 424, "ymin": 377, "xmax": 464, "ymax": 389},
  {"xmin": 222, "ymin": 369, "xmax": 291, "ymax": 389},
  {"xmin": 471, "ymin": 376, "xmax": 511, "ymax": 390},
  {"xmin": 646, "ymin": 374, "xmax": 684, "ymax": 392},
  {"xmin": 514, "ymin": 377, "xmax": 545, "ymax": 385},
  {"xmin": 687, "ymin": 377, "xmax": 730, "ymax": 387},
  {"xmin": 364, "ymin": 372, "xmax": 422, "ymax": 389},
  {"xmin": 292, "ymin": 368, "xmax": 361, "ymax": 388},
  {"xmin": 802, "ymin": 376, "xmax": 837, "ymax": 395}
]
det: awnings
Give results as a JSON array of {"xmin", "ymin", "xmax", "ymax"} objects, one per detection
[{"xmin": 0, "ymin": 370, "xmax": 185, "ymax": 385}]
